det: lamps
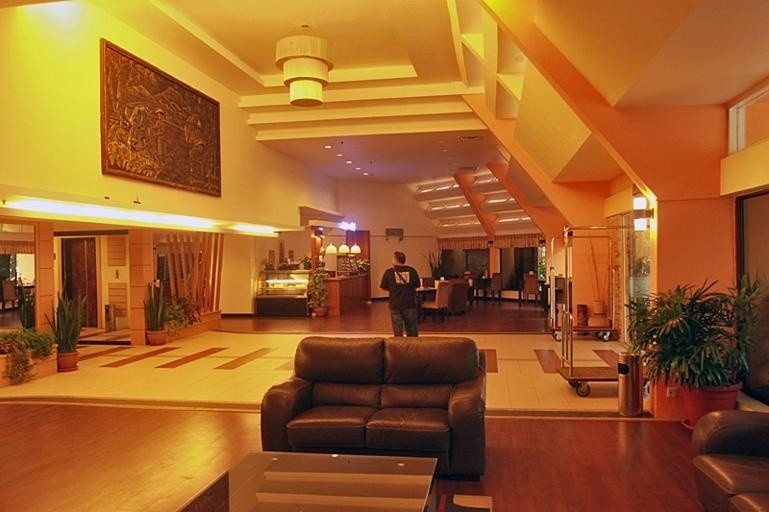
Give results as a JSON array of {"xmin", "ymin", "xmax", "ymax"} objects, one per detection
[{"xmin": 325, "ymin": 227, "xmax": 362, "ymax": 255}]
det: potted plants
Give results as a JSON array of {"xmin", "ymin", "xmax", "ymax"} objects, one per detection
[
  {"xmin": 144, "ymin": 279, "xmax": 171, "ymax": 345},
  {"xmin": 43, "ymin": 288, "xmax": 91, "ymax": 372},
  {"xmin": 307, "ymin": 264, "xmax": 331, "ymax": 317},
  {"xmin": 622, "ymin": 271, "xmax": 768, "ymax": 431}
]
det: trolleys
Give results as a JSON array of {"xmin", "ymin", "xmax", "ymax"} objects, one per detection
[{"xmin": 548, "ymin": 267, "xmax": 650, "ymax": 398}]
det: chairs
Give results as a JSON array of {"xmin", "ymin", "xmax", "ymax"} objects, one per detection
[
  {"xmin": 524, "ymin": 275, "xmax": 538, "ymax": 307},
  {"xmin": 2, "ymin": 280, "xmax": 18, "ymax": 312},
  {"xmin": 421, "ymin": 272, "xmax": 502, "ymax": 323}
]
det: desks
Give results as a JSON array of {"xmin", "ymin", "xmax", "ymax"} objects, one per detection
[
  {"xmin": 415, "ymin": 287, "xmax": 437, "ymax": 322},
  {"xmin": 519, "ymin": 280, "xmax": 546, "ymax": 307}
]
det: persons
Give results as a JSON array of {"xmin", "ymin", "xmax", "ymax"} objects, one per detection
[
  {"xmin": 380, "ymin": 251, "xmax": 420, "ymax": 337},
  {"xmin": 463, "ymin": 271, "xmax": 475, "ymax": 301}
]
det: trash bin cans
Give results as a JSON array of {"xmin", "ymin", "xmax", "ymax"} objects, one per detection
[
  {"xmin": 617, "ymin": 350, "xmax": 643, "ymax": 417},
  {"xmin": 105, "ymin": 304, "xmax": 116, "ymax": 331}
]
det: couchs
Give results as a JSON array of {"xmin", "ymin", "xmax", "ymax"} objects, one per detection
[
  {"xmin": 690, "ymin": 410, "xmax": 768, "ymax": 512},
  {"xmin": 260, "ymin": 336, "xmax": 486, "ymax": 479}
]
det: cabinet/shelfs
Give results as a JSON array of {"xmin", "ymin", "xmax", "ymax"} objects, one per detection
[{"xmin": 254, "ymin": 268, "xmax": 312, "ymax": 317}]
